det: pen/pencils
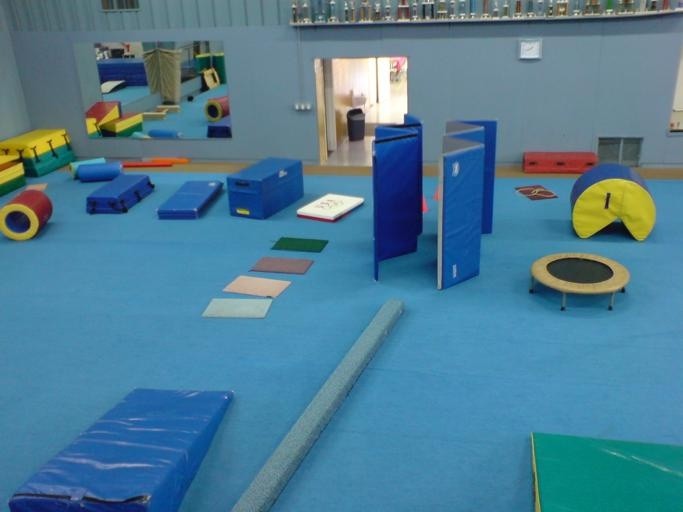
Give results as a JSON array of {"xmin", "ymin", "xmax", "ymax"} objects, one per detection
[{"xmin": 123, "ymin": 158, "xmax": 189, "ymax": 168}]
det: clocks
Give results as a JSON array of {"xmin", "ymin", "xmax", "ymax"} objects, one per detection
[{"xmin": 518, "ymin": 37, "xmax": 542, "ymax": 59}]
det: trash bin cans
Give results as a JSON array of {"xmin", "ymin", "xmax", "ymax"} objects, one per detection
[{"xmin": 347, "ymin": 108, "xmax": 365, "ymax": 140}]
